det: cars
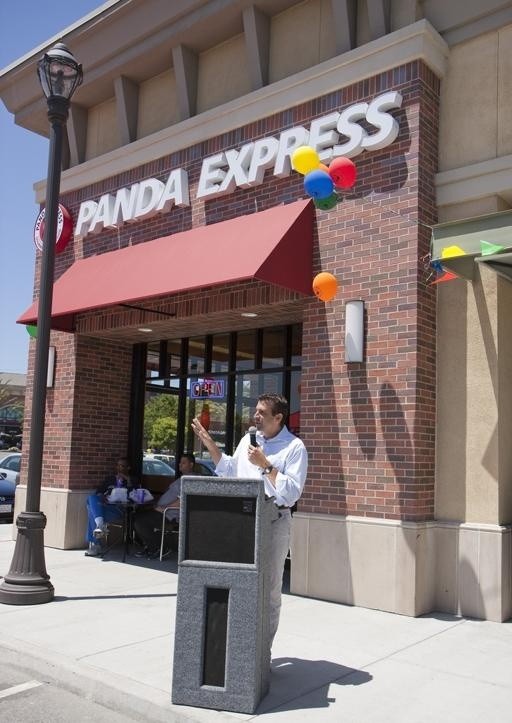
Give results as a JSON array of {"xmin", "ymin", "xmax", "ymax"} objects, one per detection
[{"xmin": 0, "ymin": 450, "xmax": 219, "ymax": 517}]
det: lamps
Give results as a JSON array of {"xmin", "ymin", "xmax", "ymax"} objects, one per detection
[
  {"xmin": 343, "ymin": 298, "xmax": 365, "ymax": 365},
  {"xmin": 45, "ymin": 345, "xmax": 56, "ymax": 390}
]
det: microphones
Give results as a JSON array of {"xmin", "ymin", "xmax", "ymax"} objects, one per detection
[{"xmin": 249, "ymin": 425, "xmax": 257, "ymax": 447}]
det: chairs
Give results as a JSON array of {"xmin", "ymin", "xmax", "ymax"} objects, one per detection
[{"xmin": 157, "ymin": 503, "xmax": 182, "ymax": 562}]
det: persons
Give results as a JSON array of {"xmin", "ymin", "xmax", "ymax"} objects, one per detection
[
  {"xmin": 131, "ymin": 453, "xmax": 198, "ymax": 562},
  {"xmin": 83, "ymin": 457, "xmax": 141, "ymax": 558},
  {"xmin": 190, "ymin": 392, "xmax": 308, "ymax": 668}
]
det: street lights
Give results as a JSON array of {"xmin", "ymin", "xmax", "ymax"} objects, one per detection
[{"xmin": 0, "ymin": 40, "xmax": 85, "ymax": 606}]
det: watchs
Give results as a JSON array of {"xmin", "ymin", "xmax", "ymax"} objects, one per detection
[{"xmin": 262, "ymin": 464, "xmax": 273, "ymax": 475}]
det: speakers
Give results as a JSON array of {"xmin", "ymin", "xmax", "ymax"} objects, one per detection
[
  {"xmin": 46, "ymin": 346, "xmax": 55, "ymax": 388},
  {"xmin": 345, "ymin": 299, "xmax": 364, "ymax": 364}
]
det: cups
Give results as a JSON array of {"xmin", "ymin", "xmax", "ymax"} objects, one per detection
[
  {"xmin": 136, "ymin": 488, "xmax": 145, "ymax": 505},
  {"xmin": 116, "ymin": 474, "xmax": 124, "ymax": 488}
]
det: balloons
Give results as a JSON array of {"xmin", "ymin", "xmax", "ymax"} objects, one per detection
[
  {"xmin": 328, "ymin": 156, "xmax": 359, "ymax": 188},
  {"xmin": 319, "ymin": 161, "xmax": 329, "ymax": 172},
  {"xmin": 304, "ymin": 167, "xmax": 333, "ymax": 198},
  {"xmin": 313, "ymin": 190, "xmax": 338, "ymax": 210},
  {"xmin": 291, "ymin": 145, "xmax": 319, "ymax": 175},
  {"xmin": 313, "ymin": 272, "xmax": 337, "ymax": 304}
]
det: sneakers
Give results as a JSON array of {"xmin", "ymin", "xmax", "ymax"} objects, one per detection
[
  {"xmin": 134, "ymin": 544, "xmax": 171, "ymax": 561},
  {"xmin": 93, "ymin": 524, "xmax": 112, "ymax": 536},
  {"xmin": 85, "ymin": 543, "xmax": 109, "ymax": 556}
]
